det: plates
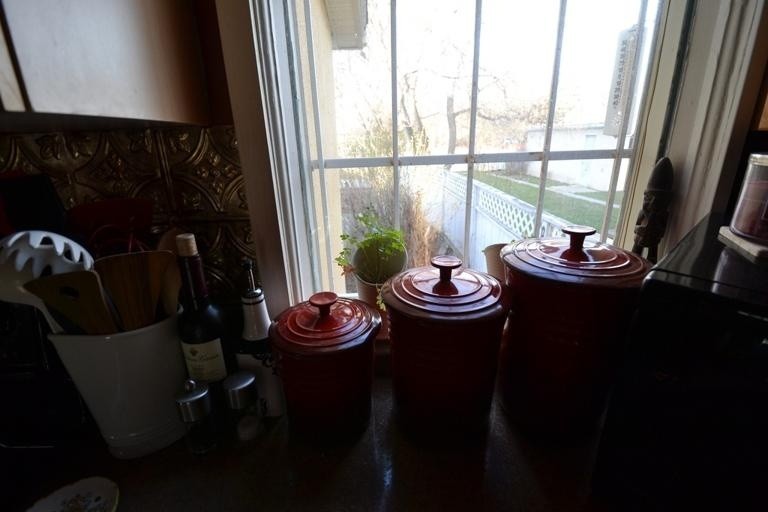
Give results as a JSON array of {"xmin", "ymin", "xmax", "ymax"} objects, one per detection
[{"xmin": 26, "ymin": 477, "xmax": 119, "ymax": 512}]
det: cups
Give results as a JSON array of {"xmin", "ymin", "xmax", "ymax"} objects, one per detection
[
  {"xmin": 730, "ymin": 153, "xmax": 768, "ymax": 238},
  {"xmin": 45, "ymin": 306, "xmax": 190, "ymax": 460}
]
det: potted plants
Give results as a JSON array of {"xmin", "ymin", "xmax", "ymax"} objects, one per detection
[{"xmin": 334, "ymin": 211, "xmax": 408, "ymax": 308}]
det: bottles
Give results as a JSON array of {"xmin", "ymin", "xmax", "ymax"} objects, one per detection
[
  {"xmin": 222, "ymin": 373, "xmax": 266, "ymax": 441},
  {"xmin": 239, "ymin": 260, "xmax": 286, "ymax": 417},
  {"xmin": 174, "ymin": 380, "xmax": 218, "ymax": 454},
  {"xmin": 711, "ymin": 246, "xmax": 745, "ymax": 298},
  {"xmin": 175, "ymin": 233, "xmax": 235, "ymax": 441}
]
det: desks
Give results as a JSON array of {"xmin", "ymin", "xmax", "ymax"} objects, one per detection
[{"xmin": 609, "ymin": 210, "xmax": 768, "ymax": 511}]
